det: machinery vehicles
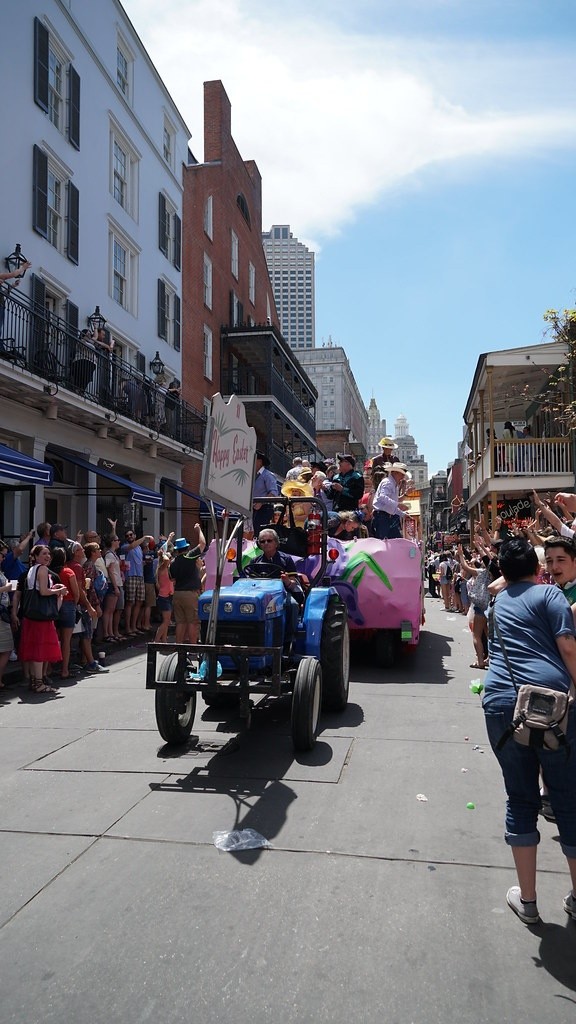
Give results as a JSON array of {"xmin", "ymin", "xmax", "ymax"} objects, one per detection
[{"xmin": 145, "ymin": 494, "xmax": 398, "ymax": 752}]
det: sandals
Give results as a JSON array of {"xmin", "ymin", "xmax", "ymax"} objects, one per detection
[
  {"xmin": 104, "ymin": 625, "xmax": 156, "ymax": 642},
  {"xmin": 17, "ymin": 670, "xmax": 81, "ymax": 693}
]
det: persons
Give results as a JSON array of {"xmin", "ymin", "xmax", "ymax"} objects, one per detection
[
  {"xmin": 248, "ymin": 528, "xmax": 298, "ymax": 591},
  {"xmin": 284, "ymin": 441, "xmax": 291, "ymax": 454},
  {"xmin": 124, "ymin": 374, "xmax": 180, "ymax": 435},
  {"xmin": 254, "ymin": 450, "xmax": 278, "ymax": 537},
  {"xmin": 282, "ymin": 455, "xmax": 365, "ymax": 541},
  {"xmin": 483, "ymin": 537, "xmax": 576, "ymax": 922},
  {"xmin": 466, "ymin": 421, "xmax": 536, "ymax": 477},
  {"xmin": 358, "ymin": 438, "xmax": 415, "ymax": 538},
  {"xmin": 425, "ymin": 489, "xmax": 576, "ymax": 669},
  {"xmin": 0, "ymin": 261, "xmax": 31, "ymax": 336},
  {"xmin": 70, "ymin": 322, "xmax": 115, "ymax": 396},
  {"xmin": 0, "ymin": 517, "xmax": 207, "ymax": 693}
]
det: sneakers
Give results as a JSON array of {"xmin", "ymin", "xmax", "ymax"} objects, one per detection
[{"xmin": 86, "ymin": 661, "xmax": 110, "ymax": 674}]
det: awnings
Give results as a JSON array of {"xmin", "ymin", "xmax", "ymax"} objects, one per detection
[
  {"xmin": 161, "ymin": 479, "xmax": 223, "ymax": 512},
  {"xmin": 0, "ymin": 444, "xmax": 54, "ymax": 485},
  {"xmin": 46, "ymin": 447, "xmax": 164, "ymax": 510}
]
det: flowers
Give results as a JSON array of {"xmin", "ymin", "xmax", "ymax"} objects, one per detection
[{"xmin": 469, "ymin": 677, "xmax": 484, "ymax": 700}]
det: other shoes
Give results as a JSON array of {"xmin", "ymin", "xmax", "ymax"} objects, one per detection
[
  {"xmin": 471, "ymin": 663, "xmax": 485, "ymax": 669},
  {"xmin": 563, "ymin": 889, "xmax": 576, "ymax": 919},
  {"xmin": 440, "ymin": 606, "xmax": 468, "ymax": 615},
  {"xmin": 507, "ymin": 886, "xmax": 539, "ymax": 923}
]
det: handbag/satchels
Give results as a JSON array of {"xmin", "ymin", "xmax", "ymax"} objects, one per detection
[
  {"xmin": 20, "ymin": 564, "xmax": 59, "ymax": 622},
  {"xmin": 510, "ymin": 684, "xmax": 569, "ymax": 749},
  {"xmin": 467, "ymin": 559, "xmax": 492, "ymax": 612}
]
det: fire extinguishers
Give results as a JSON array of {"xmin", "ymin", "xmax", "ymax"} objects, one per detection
[{"xmin": 308, "ymin": 501, "xmax": 321, "ymax": 553}]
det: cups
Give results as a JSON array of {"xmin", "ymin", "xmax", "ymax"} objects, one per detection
[
  {"xmin": 10, "ymin": 580, "xmax": 18, "ymax": 590},
  {"xmin": 85, "ymin": 578, "xmax": 91, "ymax": 589},
  {"xmin": 124, "ymin": 561, "xmax": 130, "ymax": 570}
]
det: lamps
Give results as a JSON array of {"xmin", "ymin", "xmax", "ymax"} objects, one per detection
[
  {"xmin": 97, "ymin": 426, "xmax": 108, "ymax": 439},
  {"xmin": 149, "ymin": 445, "xmax": 157, "ymax": 457},
  {"xmin": 150, "ymin": 351, "xmax": 165, "ymax": 376},
  {"xmin": 88, "ymin": 306, "xmax": 106, "ymax": 332},
  {"xmin": 5, "ymin": 244, "xmax": 29, "ymax": 278},
  {"xmin": 124, "ymin": 435, "xmax": 134, "ymax": 448},
  {"xmin": 46, "ymin": 406, "xmax": 58, "ymax": 420}
]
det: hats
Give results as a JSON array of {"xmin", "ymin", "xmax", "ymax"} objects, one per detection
[
  {"xmin": 49, "ymin": 524, "xmax": 68, "ymax": 534},
  {"xmin": 310, "ymin": 461, "xmax": 328, "ymax": 474},
  {"xmin": 173, "ymin": 538, "xmax": 189, "ymax": 549},
  {"xmin": 281, "ymin": 480, "xmax": 314, "ymax": 497},
  {"xmin": 337, "ymin": 455, "xmax": 355, "ymax": 468},
  {"xmin": 256, "ymin": 449, "xmax": 272, "ymax": 467},
  {"xmin": 378, "ymin": 438, "xmax": 398, "ymax": 449},
  {"xmin": 298, "ymin": 467, "xmax": 311, "ymax": 481},
  {"xmin": 385, "ymin": 462, "xmax": 412, "ymax": 478}
]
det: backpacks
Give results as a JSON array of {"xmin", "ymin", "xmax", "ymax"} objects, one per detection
[{"xmin": 442, "ymin": 562, "xmax": 453, "ymax": 581}]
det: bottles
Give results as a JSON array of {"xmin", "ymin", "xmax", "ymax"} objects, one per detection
[{"xmin": 110, "ymin": 339, "xmax": 115, "ymax": 349}]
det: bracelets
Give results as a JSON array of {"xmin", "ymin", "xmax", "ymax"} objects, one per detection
[
  {"xmin": 288, "ymin": 582, "xmax": 292, "ymax": 588},
  {"xmin": 404, "ymin": 494, "xmax": 407, "ymax": 496}
]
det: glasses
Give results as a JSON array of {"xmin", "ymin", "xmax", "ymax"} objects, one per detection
[
  {"xmin": 126, "ymin": 533, "xmax": 133, "ymax": 538},
  {"xmin": 260, "ymin": 539, "xmax": 274, "ymax": 544},
  {"xmin": 114, "ymin": 538, "xmax": 120, "ymax": 542},
  {"xmin": 0, "ymin": 553, "xmax": 7, "ymax": 558}
]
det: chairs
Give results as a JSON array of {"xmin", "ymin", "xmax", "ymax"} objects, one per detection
[
  {"xmin": 98, "ymin": 386, "xmax": 129, "ymax": 412},
  {"xmin": 35, "ymin": 350, "xmax": 69, "ymax": 383},
  {"xmin": 0, "ymin": 337, "xmax": 26, "ymax": 368}
]
